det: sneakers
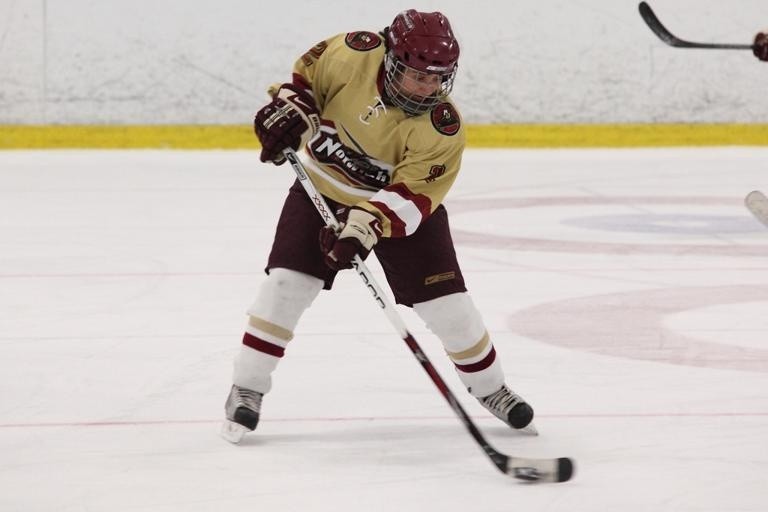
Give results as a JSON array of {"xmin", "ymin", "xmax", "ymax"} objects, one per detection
[
  {"xmin": 225, "ymin": 384, "xmax": 263, "ymax": 430},
  {"xmin": 477, "ymin": 384, "xmax": 533, "ymax": 429}
]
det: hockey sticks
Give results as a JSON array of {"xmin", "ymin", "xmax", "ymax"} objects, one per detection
[
  {"xmin": 745, "ymin": 190, "xmax": 768, "ymax": 225},
  {"xmin": 637, "ymin": 1, "xmax": 753, "ymax": 50},
  {"xmin": 282, "ymin": 146, "xmax": 573, "ymax": 483}
]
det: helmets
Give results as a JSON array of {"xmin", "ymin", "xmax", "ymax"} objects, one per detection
[{"xmin": 379, "ymin": 10, "xmax": 459, "ymax": 115}]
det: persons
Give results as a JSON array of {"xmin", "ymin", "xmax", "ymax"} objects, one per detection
[{"xmin": 223, "ymin": 6, "xmax": 535, "ymax": 432}]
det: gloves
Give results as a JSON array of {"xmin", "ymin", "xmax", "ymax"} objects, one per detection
[
  {"xmin": 320, "ymin": 206, "xmax": 383, "ymax": 272},
  {"xmin": 255, "ymin": 83, "xmax": 321, "ymax": 165}
]
